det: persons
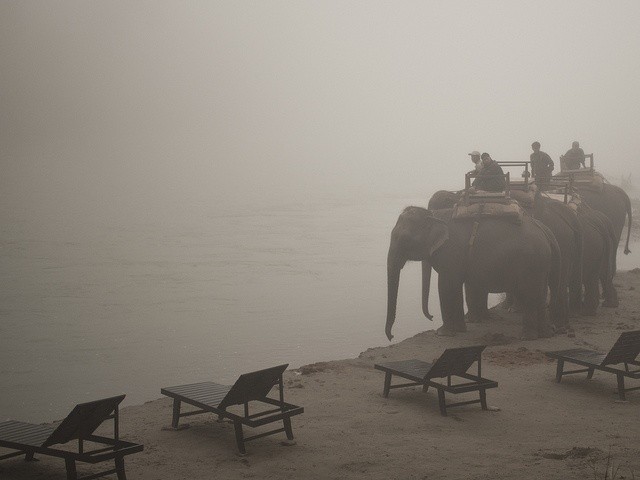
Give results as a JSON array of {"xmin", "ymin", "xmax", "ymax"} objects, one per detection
[
  {"xmin": 471, "ymin": 152, "xmax": 507, "ymax": 194},
  {"xmin": 561, "ymin": 140, "xmax": 586, "ymax": 171},
  {"xmin": 530, "ymin": 141, "xmax": 555, "ymax": 188},
  {"xmin": 468, "ymin": 150, "xmax": 486, "ymax": 187}
]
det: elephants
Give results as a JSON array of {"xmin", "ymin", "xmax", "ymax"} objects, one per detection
[
  {"xmin": 385, "ymin": 204, "xmax": 562, "ymax": 341},
  {"xmin": 422, "ymin": 189, "xmax": 583, "ymax": 332},
  {"xmin": 553, "ymin": 171, "xmax": 632, "ymax": 256},
  {"xmin": 514, "ymin": 184, "xmax": 616, "ymax": 318}
]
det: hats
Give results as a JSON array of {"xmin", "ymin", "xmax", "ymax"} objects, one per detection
[{"xmin": 469, "ymin": 151, "xmax": 480, "ymax": 156}]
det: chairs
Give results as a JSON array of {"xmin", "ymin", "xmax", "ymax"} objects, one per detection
[
  {"xmin": 161, "ymin": 364, "xmax": 303, "ymax": 453},
  {"xmin": 0, "ymin": 394, "xmax": 144, "ymax": 480},
  {"xmin": 545, "ymin": 331, "xmax": 640, "ymax": 399},
  {"xmin": 373, "ymin": 345, "xmax": 498, "ymax": 414}
]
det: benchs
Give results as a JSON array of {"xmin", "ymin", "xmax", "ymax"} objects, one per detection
[
  {"xmin": 465, "ymin": 172, "xmax": 510, "ymax": 203},
  {"xmin": 560, "ymin": 155, "xmax": 594, "ymax": 176},
  {"xmin": 537, "ymin": 175, "xmax": 575, "ymax": 203},
  {"xmin": 495, "ymin": 161, "xmax": 536, "ymax": 188}
]
